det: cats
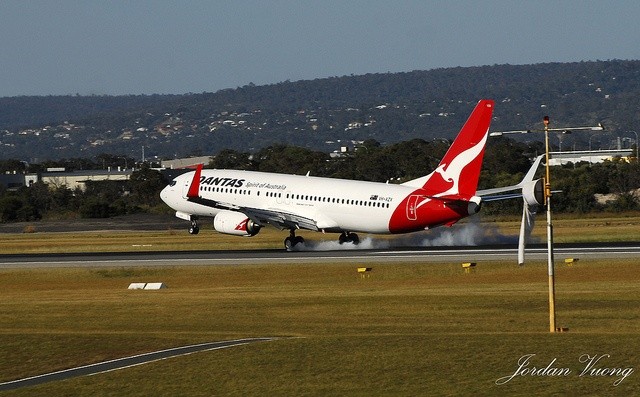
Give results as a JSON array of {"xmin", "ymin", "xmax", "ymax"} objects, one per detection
[{"xmin": 159, "ymin": 98, "xmax": 547, "ymax": 251}]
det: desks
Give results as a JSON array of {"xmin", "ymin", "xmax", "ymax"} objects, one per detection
[{"xmin": 489, "ymin": 115, "xmax": 605, "ymax": 333}]
